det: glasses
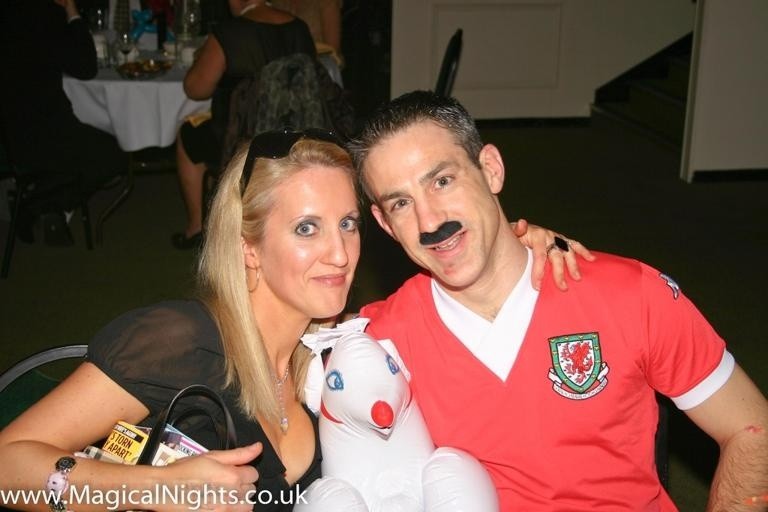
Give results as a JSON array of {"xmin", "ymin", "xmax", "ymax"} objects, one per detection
[{"xmin": 240, "ymin": 127, "xmax": 350, "ymax": 199}]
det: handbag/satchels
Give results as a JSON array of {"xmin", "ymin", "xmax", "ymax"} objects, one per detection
[{"xmin": 72, "ymin": 383, "xmax": 238, "ymax": 467}]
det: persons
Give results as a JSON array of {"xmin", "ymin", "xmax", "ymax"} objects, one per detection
[
  {"xmin": 0, "ymin": 122, "xmax": 598, "ymax": 511},
  {"xmin": 2, "ymin": 0, "xmax": 122, "ymax": 245},
  {"xmin": 324, "ymin": 89, "xmax": 767, "ymax": 512},
  {"xmin": 168, "ymin": 0, "xmax": 342, "ymax": 250},
  {"xmin": 289, "ymin": 317, "xmax": 499, "ymax": 512}
]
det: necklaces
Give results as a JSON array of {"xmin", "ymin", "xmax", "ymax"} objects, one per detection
[
  {"xmin": 274, "ymin": 364, "xmax": 290, "ymax": 434},
  {"xmin": 240, "ymin": 1, "xmax": 272, "ymax": 17}
]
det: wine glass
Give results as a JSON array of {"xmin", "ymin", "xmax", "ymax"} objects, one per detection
[{"xmin": 117, "ymin": 32, "xmax": 135, "ymax": 72}]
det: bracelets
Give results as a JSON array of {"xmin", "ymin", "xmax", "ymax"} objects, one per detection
[{"xmin": 45, "ymin": 455, "xmax": 77, "ymax": 511}]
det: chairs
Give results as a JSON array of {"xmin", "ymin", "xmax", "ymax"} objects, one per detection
[{"xmin": 419, "ymin": 27, "xmax": 475, "ymax": 97}]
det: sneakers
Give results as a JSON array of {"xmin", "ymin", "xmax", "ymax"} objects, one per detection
[
  {"xmin": 44, "ymin": 205, "xmax": 69, "ymax": 247},
  {"xmin": 7, "ymin": 200, "xmax": 35, "ymax": 245}
]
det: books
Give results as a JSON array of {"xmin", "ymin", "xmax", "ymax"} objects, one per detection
[{"xmin": 74, "ymin": 420, "xmax": 211, "ymax": 468}]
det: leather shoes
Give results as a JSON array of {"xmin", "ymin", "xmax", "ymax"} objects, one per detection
[{"xmin": 170, "ymin": 231, "xmax": 203, "ymax": 249}]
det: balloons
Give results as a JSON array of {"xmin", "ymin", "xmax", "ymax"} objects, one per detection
[{"xmin": 128, "ymin": 9, "xmax": 160, "ymax": 41}]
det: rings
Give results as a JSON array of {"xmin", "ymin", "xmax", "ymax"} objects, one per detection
[{"xmin": 546, "ymin": 236, "xmax": 569, "ymax": 256}]
